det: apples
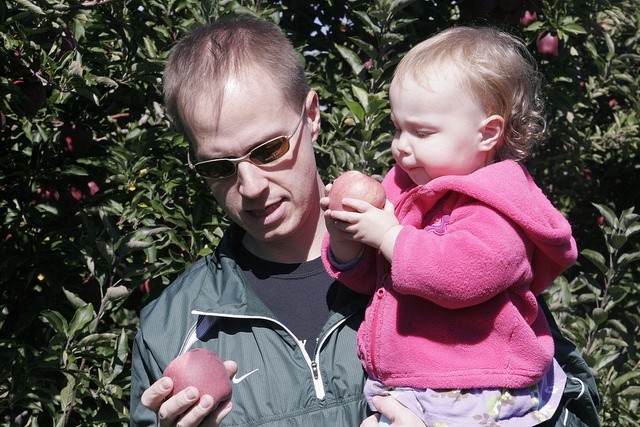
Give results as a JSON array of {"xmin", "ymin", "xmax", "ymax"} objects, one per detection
[
  {"xmin": 30, "ymin": 186, "xmax": 59, "ymax": 208},
  {"xmin": 369, "ymin": 32, "xmax": 376, "ymax": 38},
  {"xmin": 595, "ymin": 216, "xmax": 606, "ymax": 225},
  {"xmin": 365, "ymin": 56, "xmax": 373, "ymax": 70},
  {"xmin": 64, "ymin": 137, "xmax": 74, "ymax": 150},
  {"xmin": 161, "ymin": 348, "xmax": 232, "ymax": 414},
  {"xmin": 11, "ymin": 76, "xmax": 25, "ymax": 90},
  {"xmin": 327, "ymin": 171, "xmax": 385, "ymax": 214},
  {"xmin": 139, "ymin": 277, "xmax": 151, "ymax": 293},
  {"xmin": 609, "ymin": 97, "xmax": 619, "ymax": 110},
  {"xmin": 578, "ymin": 79, "xmax": 586, "ymax": 89},
  {"xmin": 601, "ymin": 18, "xmax": 614, "ymax": 31},
  {"xmin": 12, "ymin": 50, "xmax": 20, "ymax": 58},
  {"xmin": 70, "ymin": 187, "xmax": 81, "ymax": 198},
  {"xmin": 536, "ymin": 32, "xmax": 557, "ymax": 57},
  {"xmin": 339, "ymin": 25, "xmax": 346, "ymax": 32},
  {"xmin": 85, "ymin": 179, "xmax": 98, "ymax": 197},
  {"xmin": 6, "ymin": 232, "xmax": 12, "ymax": 242},
  {"xmin": 522, "ymin": 9, "xmax": 538, "ymax": 25}
]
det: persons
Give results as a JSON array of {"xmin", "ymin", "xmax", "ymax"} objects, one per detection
[
  {"xmin": 126, "ymin": 13, "xmax": 377, "ymax": 425},
  {"xmin": 319, "ymin": 28, "xmax": 577, "ymax": 425}
]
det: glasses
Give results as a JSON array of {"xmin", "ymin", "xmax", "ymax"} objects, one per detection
[{"xmin": 185, "ymin": 100, "xmax": 305, "ymax": 182}]
